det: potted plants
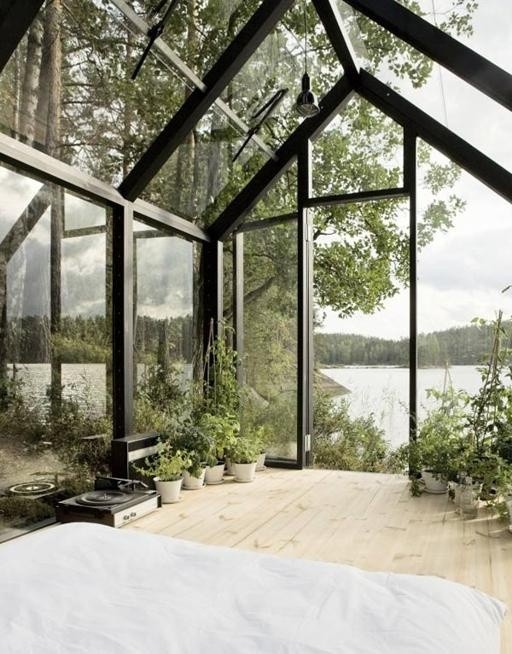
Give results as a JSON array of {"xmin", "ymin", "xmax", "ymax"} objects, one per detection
[
  {"xmin": 131, "ymin": 311, "xmax": 271, "ymax": 504},
  {"xmin": 389, "ymin": 317, "xmax": 512, "ymax": 520}
]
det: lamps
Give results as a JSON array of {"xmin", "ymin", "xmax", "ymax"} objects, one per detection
[{"xmin": 296, "ymin": 0, "xmax": 321, "ymax": 118}]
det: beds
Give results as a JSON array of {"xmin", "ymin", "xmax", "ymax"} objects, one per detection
[{"xmin": 0, "ymin": 521, "xmax": 506, "ymax": 654}]
